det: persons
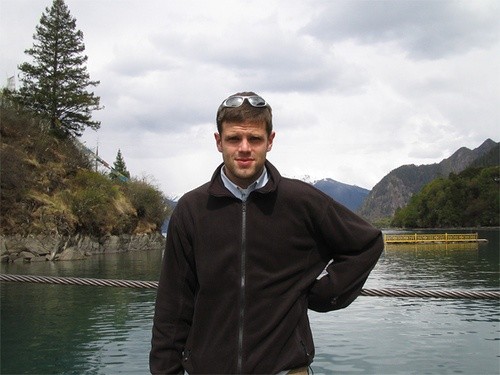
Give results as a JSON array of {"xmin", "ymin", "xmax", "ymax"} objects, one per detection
[{"xmin": 149, "ymin": 92, "xmax": 384, "ymax": 375}]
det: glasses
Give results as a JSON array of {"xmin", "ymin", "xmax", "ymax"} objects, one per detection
[{"xmin": 216, "ymin": 95, "xmax": 271, "ymax": 121}]
